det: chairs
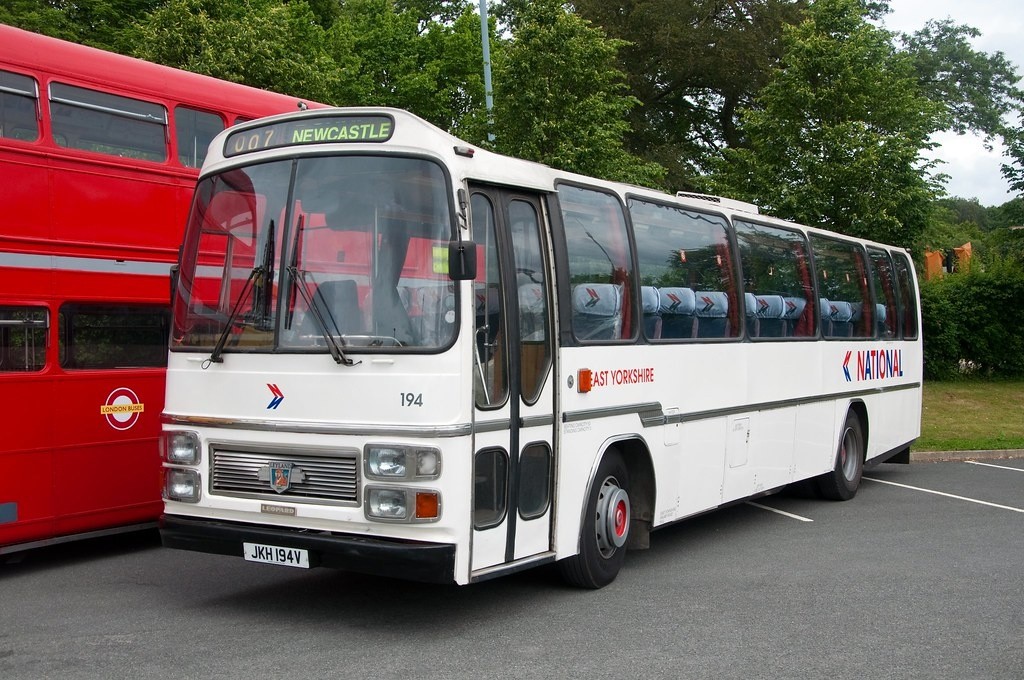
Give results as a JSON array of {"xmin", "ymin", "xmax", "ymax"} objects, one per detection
[
  {"xmin": 9, "ymin": 125, "xmax": 70, "ymax": 148},
  {"xmin": 297, "ymin": 279, "xmax": 363, "ymax": 337},
  {"xmin": 475, "ymin": 281, "xmax": 885, "ymax": 342}
]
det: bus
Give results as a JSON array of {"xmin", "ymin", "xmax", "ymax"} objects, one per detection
[
  {"xmin": 0, "ymin": 22, "xmax": 334, "ymax": 556},
  {"xmin": 158, "ymin": 102, "xmax": 923, "ymax": 590}
]
